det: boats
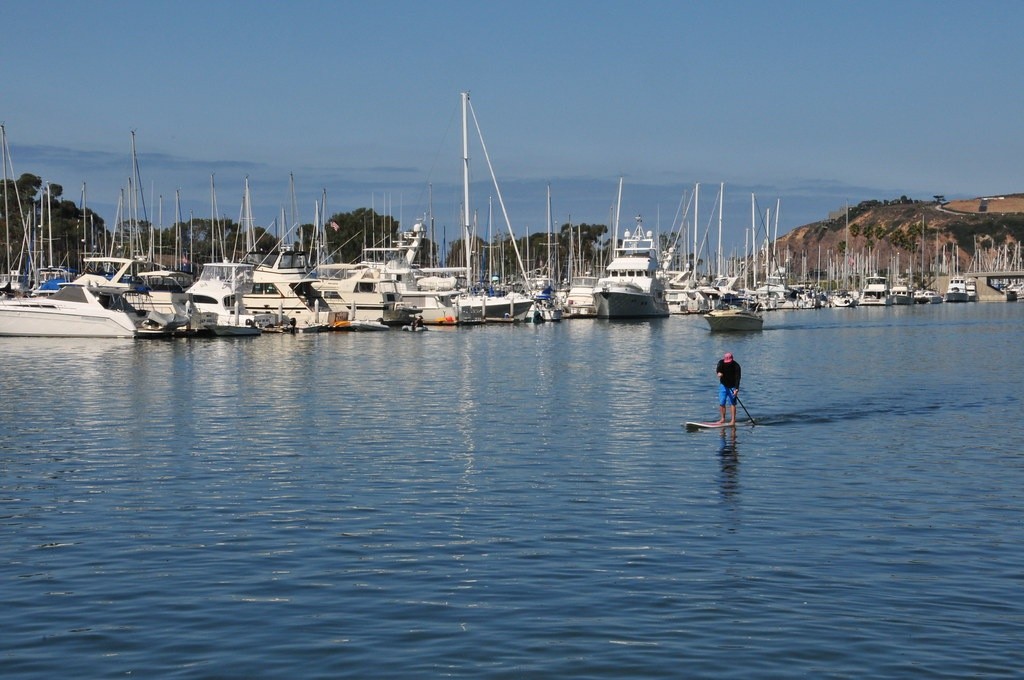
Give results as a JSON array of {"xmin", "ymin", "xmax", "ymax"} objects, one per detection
[
  {"xmin": 589, "ymin": 215, "xmax": 672, "ymax": 317},
  {"xmin": 702, "ymin": 296, "xmax": 767, "ymax": 333}
]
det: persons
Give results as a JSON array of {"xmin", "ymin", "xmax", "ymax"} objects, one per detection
[{"xmin": 716, "ymin": 352, "xmax": 744, "ymax": 426}]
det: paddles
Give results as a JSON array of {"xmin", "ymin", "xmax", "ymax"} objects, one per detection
[{"xmin": 721, "ymin": 375, "xmax": 756, "ymax": 426}]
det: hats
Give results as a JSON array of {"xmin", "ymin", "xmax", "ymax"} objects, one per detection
[{"xmin": 724, "ymin": 353, "xmax": 732, "ymax": 363}]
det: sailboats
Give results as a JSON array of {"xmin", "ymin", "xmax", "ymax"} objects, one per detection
[{"xmin": 0, "ymin": 86, "xmax": 1023, "ymax": 338}]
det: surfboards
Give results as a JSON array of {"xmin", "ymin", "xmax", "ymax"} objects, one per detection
[{"xmin": 686, "ymin": 420, "xmax": 754, "ymax": 427}]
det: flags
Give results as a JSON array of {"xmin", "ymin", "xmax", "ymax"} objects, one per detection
[{"xmin": 331, "ymin": 222, "xmax": 339, "ymax": 231}]
